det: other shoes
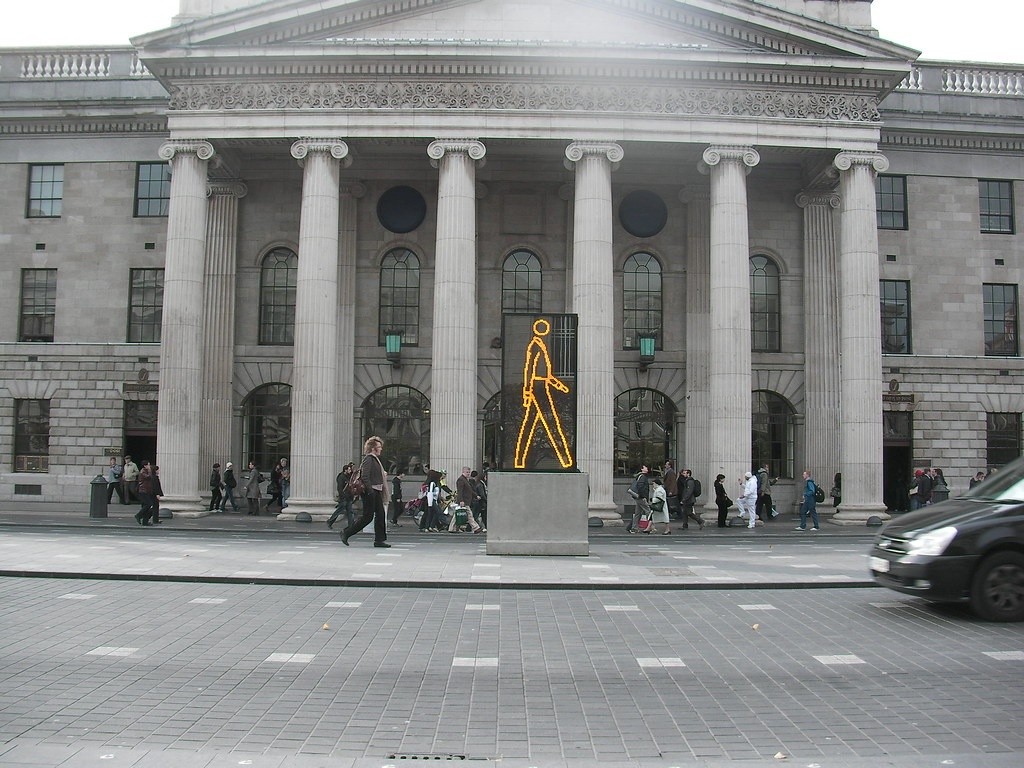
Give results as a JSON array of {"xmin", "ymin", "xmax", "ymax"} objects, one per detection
[
  {"xmin": 135, "ymin": 505, "xmax": 487, "ymax": 533},
  {"xmin": 374, "ymin": 541, "xmax": 392, "ymax": 547},
  {"xmin": 339, "ymin": 531, "xmax": 349, "ymax": 546},
  {"xmin": 629, "ymin": 511, "xmax": 819, "ymax": 535}
]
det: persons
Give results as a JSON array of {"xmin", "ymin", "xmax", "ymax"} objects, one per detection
[
  {"xmin": 735, "ymin": 464, "xmax": 780, "ymax": 528},
  {"xmin": 663, "ymin": 461, "xmax": 707, "ymax": 531},
  {"xmin": 418, "ymin": 462, "xmax": 494, "ymax": 532},
  {"xmin": 628, "ymin": 465, "xmax": 657, "ymax": 534},
  {"xmin": 833, "ymin": 473, "xmax": 841, "ymax": 507},
  {"xmin": 642, "ymin": 478, "xmax": 672, "ymax": 535},
  {"xmin": 326, "ymin": 462, "xmax": 356, "ymax": 529},
  {"xmin": 339, "ymin": 436, "xmax": 392, "ymax": 548},
  {"xmin": 390, "ymin": 469, "xmax": 406, "ymax": 527},
  {"xmin": 220, "ymin": 462, "xmax": 241, "ymax": 511},
  {"xmin": 713, "ymin": 473, "xmax": 732, "ymax": 527},
  {"xmin": 122, "ymin": 455, "xmax": 142, "ymax": 505},
  {"xmin": 135, "ymin": 460, "xmax": 164, "ymax": 526},
  {"xmin": 263, "ymin": 458, "xmax": 290, "ymax": 511},
  {"xmin": 244, "ymin": 461, "xmax": 261, "ymax": 515},
  {"xmin": 895, "ymin": 467, "xmax": 948, "ymax": 513},
  {"xmin": 794, "ymin": 470, "xmax": 820, "ymax": 530},
  {"xmin": 969, "ymin": 466, "xmax": 999, "ymax": 493},
  {"xmin": 210, "ymin": 462, "xmax": 223, "ymax": 511},
  {"xmin": 106, "ymin": 457, "xmax": 126, "ymax": 504}
]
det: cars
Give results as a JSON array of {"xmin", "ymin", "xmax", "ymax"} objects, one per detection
[{"xmin": 867, "ymin": 449, "xmax": 1024, "ymax": 624}]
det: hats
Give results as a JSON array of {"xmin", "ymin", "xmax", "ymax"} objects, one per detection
[
  {"xmin": 745, "ymin": 471, "xmax": 752, "ymax": 479},
  {"xmin": 914, "ymin": 470, "xmax": 921, "ymax": 476}
]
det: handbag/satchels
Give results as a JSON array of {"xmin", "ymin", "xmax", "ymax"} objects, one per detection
[
  {"xmin": 426, "ymin": 481, "xmax": 436, "ymax": 507},
  {"xmin": 723, "ymin": 496, "xmax": 734, "ymax": 509},
  {"xmin": 454, "ymin": 506, "xmax": 469, "ymax": 526},
  {"xmin": 257, "ymin": 473, "xmax": 265, "ymax": 483},
  {"xmin": 626, "ymin": 474, "xmax": 644, "ymax": 500},
  {"xmin": 648, "ymin": 497, "xmax": 665, "ymax": 513},
  {"xmin": 830, "ymin": 487, "xmax": 841, "ymax": 498},
  {"xmin": 334, "ymin": 493, "xmax": 342, "ymax": 502},
  {"xmin": 266, "ymin": 480, "xmax": 280, "ymax": 494},
  {"xmin": 112, "ymin": 464, "xmax": 123, "ymax": 478},
  {"xmin": 909, "ymin": 479, "xmax": 919, "ymax": 495},
  {"xmin": 349, "ymin": 463, "xmax": 366, "ymax": 495}
]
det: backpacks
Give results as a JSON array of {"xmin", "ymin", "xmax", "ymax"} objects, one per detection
[
  {"xmin": 805, "ymin": 480, "xmax": 825, "ymax": 503},
  {"xmin": 685, "ymin": 476, "xmax": 702, "ymax": 497}
]
